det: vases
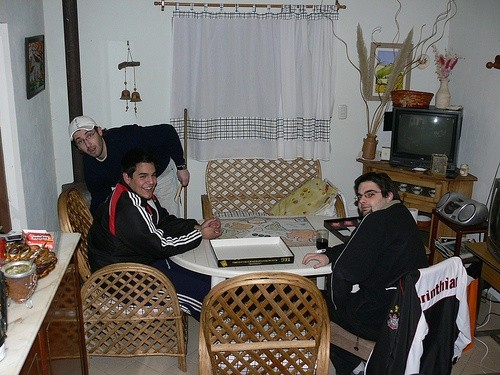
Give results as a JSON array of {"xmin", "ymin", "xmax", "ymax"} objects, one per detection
[
  {"xmin": 361, "ymin": 136, "xmax": 377, "ymax": 160},
  {"xmin": 435, "ymin": 80, "xmax": 450, "ymax": 109}
]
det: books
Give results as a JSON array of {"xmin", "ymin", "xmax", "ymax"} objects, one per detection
[{"xmin": 435, "ymin": 236, "xmax": 476, "ymax": 260}]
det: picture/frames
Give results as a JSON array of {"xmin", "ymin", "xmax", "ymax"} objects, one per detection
[
  {"xmin": 368, "ymin": 42, "xmax": 412, "ymax": 101},
  {"xmin": 25, "ymin": 35, "xmax": 46, "ymax": 99}
]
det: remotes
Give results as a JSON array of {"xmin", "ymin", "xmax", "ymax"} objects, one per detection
[{"xmin": 446, "ymin": 171, "xmax": 458, "ymax": 180}]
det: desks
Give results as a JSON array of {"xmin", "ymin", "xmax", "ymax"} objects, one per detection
[
  {"xmin": 361, "ymin": 161, "xmax": 477, "ymax": 266},
  {"xmin": 429, "ymin": 209, "xmax": 488, "ymax": 267},
  {"xmin": 169, "ymin": 214, "xmax": 342, "ymax": 278},
  {"xmin": 0, "ymin": 234, "xmax": 88, "ymax": 375}
]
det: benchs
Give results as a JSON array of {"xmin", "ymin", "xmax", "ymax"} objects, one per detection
[{"xmin": 200, "ymin": 158, "xmax": 346, "ymax": 222}]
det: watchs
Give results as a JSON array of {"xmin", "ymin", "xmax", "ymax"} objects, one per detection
[{"xmin": 177, "ymin": 164, "xmax": 187, "ymax": 169}]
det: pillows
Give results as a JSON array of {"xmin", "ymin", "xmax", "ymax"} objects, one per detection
[{"xmin": 269, "ymin": 178, "xmax": 334, "ymax": 217}]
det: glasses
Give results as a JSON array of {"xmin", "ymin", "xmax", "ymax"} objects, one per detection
[
  {"xmin": 77, "ymin": 131, "xmax": 96, "ymax": 145},
  {"xmin": 354, "ymin": 191, "xmax": 381, "ymax": 201}
]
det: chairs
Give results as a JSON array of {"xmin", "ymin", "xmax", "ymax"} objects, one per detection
[{"xmin": 56, "ymin": 158, "xmax": 471, "ymax": 375}]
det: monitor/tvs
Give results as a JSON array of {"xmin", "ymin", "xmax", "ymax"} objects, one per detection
[
  {"xmin": 389, "ymin": 106, "xmax": 462, "ymax": 172},
  {"xmin": 486, "ymin": 178, "xmax": 500, "ymax": 262}
]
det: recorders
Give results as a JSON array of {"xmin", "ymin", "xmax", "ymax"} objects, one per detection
[{"xmin": 435, "ymin": 163, "xmax": 500, "ymax": 227}]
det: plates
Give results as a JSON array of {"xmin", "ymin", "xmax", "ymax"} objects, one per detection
[
  {"xmin": 0, "ymin": 245, "xmax": 57, "ymax": 278},
  {"xmin": 414, "ymin": 168, "xmax": 427, "ymax": 173}
]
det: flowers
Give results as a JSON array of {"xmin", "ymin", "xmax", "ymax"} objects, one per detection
[{"xmin": 434, "ymin": 54, "xmax": 458, "ymax": 77}]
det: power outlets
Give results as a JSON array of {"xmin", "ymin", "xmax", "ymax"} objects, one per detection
[{"xmin": 339, "ymin": 105, "xmax": 347, "ymax": 119}]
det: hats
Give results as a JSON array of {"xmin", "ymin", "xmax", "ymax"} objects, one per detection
[{"xmin": 69, "ymin": 115, "xmax": 98, "ymax": 141}]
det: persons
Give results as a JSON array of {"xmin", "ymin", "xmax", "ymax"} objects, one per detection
[
  {"xmin": 68, "ymin": 115, "xmax": 189, "ymax": 218},
  {"xmin": 87, "ymin": 153, "xmax": 223, "ymax": 322},
  {"xmin": 303, "ymin": 171, "xmax": 427, "ymax": 375}
]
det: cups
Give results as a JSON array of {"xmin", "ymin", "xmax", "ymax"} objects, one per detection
[
  {"xmin": 428, "ymin": 190, "xmax": 435, "ymax": 198},
  {"xmin": 460, "ymin": 164, "xmax": 468, "ymax": 176},
  {"xmin": 431, "ymin": 155, "xmax": 448, "ymax": 177},
  {"xmin": 413, "ymin": 186, "xmax": 422, "ymax": 194},
  {"xmin": 400, "ymin": 183, "xmax": 407, "ymax": 192},
  {"xmin": 316, "ymin": 229, "xmax": 329, "ymax": 250},
  {"xmin": 3, "ymin": 260, "xmax": 37, "ymax": 302}
]
